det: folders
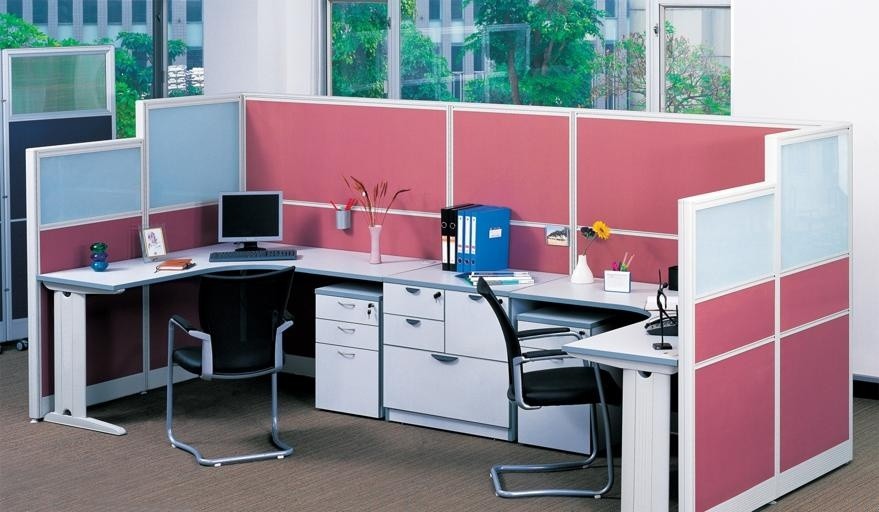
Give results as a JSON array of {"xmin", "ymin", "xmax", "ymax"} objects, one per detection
[{"xmin": 441, "ymin": 203, "xmax": 510, "ymax": 272}]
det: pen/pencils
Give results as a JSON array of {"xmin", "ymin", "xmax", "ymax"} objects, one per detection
[
  {"xmin": 330, "ymin": 198, "xmax": 355, "ymax": 211},
  {"xmin": 611, "ymin": 252, "xmax": 635, "ymax": 271}
]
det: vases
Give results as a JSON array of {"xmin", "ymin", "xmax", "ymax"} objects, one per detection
[
  {"xmin": 571, "ymin": 255, "xmax": 594, "ymax": 285},
  {"xmin": 367, "ymin": 225, "xmax": 382, "ymax": 266}
]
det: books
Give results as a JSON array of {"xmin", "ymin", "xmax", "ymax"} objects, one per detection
[
  {"xmin": 153, "ymin": 258, "xmax": 196, "ymax": 274},
  {"xmin": 468, "ymin": 269, "xmax": 536, "ymax": 286}
]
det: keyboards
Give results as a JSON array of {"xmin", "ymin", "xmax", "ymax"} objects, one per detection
[{"xmin": 209, "ymin": 249, "xmax": 297, "ymax": 262}]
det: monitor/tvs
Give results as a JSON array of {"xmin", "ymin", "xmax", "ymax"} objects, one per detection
[{"xmin": 218, "ymin": 191, "xmax": 283, "ymax": 251}]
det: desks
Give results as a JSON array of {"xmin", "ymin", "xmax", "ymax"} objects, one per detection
[
  {"xmin": 380, "ymin": 262, "xmax": 681, "ymax": 512},
  {"xmin": 37, "ymin": 238, "xmax": 443, "ymax": 511}
]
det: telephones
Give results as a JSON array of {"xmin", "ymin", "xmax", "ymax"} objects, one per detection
[{"xmin": 645, "ymin": 313, "xmax": 678, "ymax": 336}]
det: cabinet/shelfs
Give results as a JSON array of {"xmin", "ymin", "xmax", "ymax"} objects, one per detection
[
  {"xmin": 307, "ymin": 284, "xmax": 383, "ymax": 422},
  {"xmin": 516, "ymin": 308, "xmax": 614, "ymax": 456}
]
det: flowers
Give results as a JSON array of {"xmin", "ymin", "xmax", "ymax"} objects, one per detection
[
  {"xmin": 581, "ymin": 221, "xmax": 610, "ymax": 255},
  {"xmin": 344, "ymin": 176, "xmax": 412, "ymax": 227}
]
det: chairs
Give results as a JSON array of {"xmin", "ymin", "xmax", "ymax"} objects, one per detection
[
  {"xmin": 165, "ymin": 269, "xmax": 299, "ymax": 467},
  {"xmin": 475, "ymin": 276, "xmax": 617, "ymax": 499}
]
